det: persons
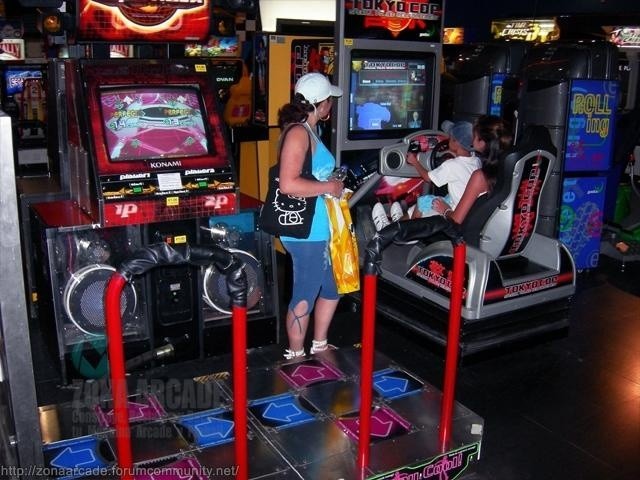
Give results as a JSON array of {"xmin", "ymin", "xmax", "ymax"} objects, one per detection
[
  {"xmin": 367, "ymin": 115, "xmax": 515, "ymax": 245},
  {"xmin": 257, "ymin": 69, "xmax": 346, "ymax": 361},
  {"xmin": 398, "ymin": 120, "xmax": 490, "ymax": 222}
]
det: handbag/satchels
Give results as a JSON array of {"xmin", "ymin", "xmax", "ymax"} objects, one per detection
[{"xmin": 261, "ymin": 165, "xmax": 319, "ymax": 239}]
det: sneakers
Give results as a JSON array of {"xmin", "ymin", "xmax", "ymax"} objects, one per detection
[
  {"xmin": 391, "ymin": 202, "xmax": 404, "ymax": 222},
  {"xmin": 373, "ymin": 202, "xmax": 391, "ymax": 231},
  {"xmin": 310, "ymin": 344, "xmax": 338, "ymax": 354}
]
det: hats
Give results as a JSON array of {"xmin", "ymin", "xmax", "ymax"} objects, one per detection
[
  {"xmin": 295, "ymin": 72, "xmax": 343, "ymax": 104},
  {"xmin": 442, "ymin": 119, "xmax": 473, "ymax": 150}
]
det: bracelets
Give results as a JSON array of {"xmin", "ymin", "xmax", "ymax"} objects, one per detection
[{"xmin": 443, "ymin": 208, "xmax": 453, "ymax": 224}]
